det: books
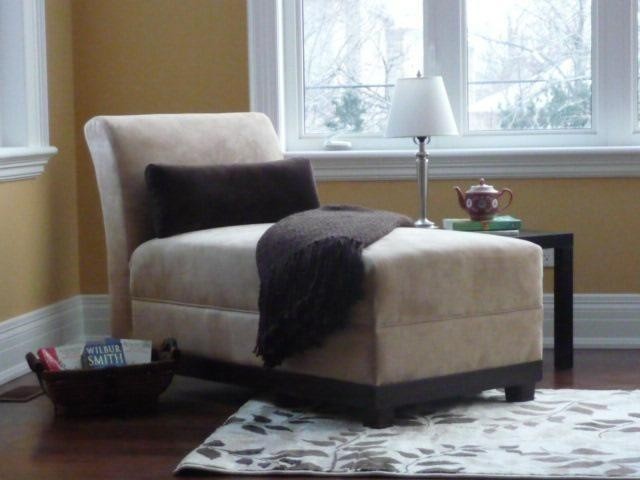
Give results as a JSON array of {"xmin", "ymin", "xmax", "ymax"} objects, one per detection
[
  {"xmin": 37, "ymin": 337, "xmax": 153, "ymax": 371},
  {"xmin": 471, "ymin": 229, "xmax": 520, "ymax": 238},
  {"xmin": 442, "ymin": 215, "xmax": 522, "ymax": 231}
]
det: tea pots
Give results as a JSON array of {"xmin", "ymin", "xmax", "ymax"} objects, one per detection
[{"xmin": 454, "ymin": 178, "xmax": 512, "ymax": 220}]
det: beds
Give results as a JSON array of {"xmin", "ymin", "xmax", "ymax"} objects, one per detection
[{"xmin": 82, "ymin": 111, "xmax": 545, "ymax": 429}]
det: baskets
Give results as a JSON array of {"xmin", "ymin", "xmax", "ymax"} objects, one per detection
[{"xmin": 24, "ymin": 335, "xmax": 182, "ymax": 416}]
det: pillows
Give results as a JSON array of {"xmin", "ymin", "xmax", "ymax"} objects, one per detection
[{"xmin": 144, "ymin": 155, "xmax": 320, "ymax": 239}]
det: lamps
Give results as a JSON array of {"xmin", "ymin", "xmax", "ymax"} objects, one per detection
[{"xmin": 382, "ymin": 69, "xmax": 461, "ymax": 230}]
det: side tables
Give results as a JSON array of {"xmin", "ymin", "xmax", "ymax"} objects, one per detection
[{"xmin": 502, "ymin": 229, "xmax": 576, "ymax": 369}]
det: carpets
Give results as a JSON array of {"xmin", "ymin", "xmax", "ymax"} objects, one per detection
[{"xmin": 171, "ymin": 387, "xmax": 640, "ymax": 480}]
